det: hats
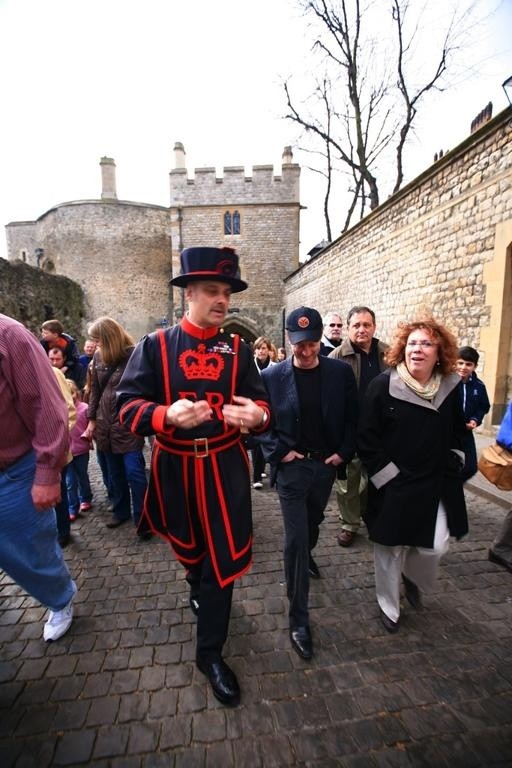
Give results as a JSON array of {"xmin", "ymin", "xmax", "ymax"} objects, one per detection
[
  {"xmin": 169, "ymin": 247, "xmax": 248, "ymax": 293},
  {"xmin": 285, "ymin": 305, "xmax": 324, "ymax": 345}
]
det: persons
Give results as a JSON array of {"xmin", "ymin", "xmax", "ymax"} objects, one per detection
[
  {"xmin": 478, "ymin": 399, "xmax": 512, "ymax": 571},
  {"xmin": 1, "ymin": 314, "xmax": 78, "ymax": 643},
  {"xmin": 39, "ymin": 306, "xmax": 489, "ymax": 548},
  {"xmin": 359, "ymin": 317, "xmax": 477, "ymax": 634},
  {"xmin": 259, "ymin": 307, "xmax": 358, "ymax": 660},
  {"xmin": 117, "ymin": 247, "xmax": 271, "ymax": 709}
]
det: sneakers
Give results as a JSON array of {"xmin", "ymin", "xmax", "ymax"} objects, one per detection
[
  {"xmin": 56, "ymin": 498, "xmax": 152, "ymax": 550},
  {"xmin": 43, "ymin": 580, "xmax": 77, "ymax": 642},
  {"xmin": 337, "ymin": 529, "xmax": 354, "ymax": 548},
  {"xmin": 254, "ymin": 482, "xmax": 264, "ymax": 490}
]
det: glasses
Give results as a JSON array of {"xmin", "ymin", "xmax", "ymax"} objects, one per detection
[{"xmin": 324, "ymin": 324, "xmax": 343, "ymax": 327}]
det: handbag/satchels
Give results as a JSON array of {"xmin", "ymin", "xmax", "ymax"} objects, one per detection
[{"xmin": 478, "ymin": 441, "xmax": 512, "ymax": 490}]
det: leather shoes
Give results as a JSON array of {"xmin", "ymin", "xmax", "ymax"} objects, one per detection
[
  {"xmin": 308, "ymin": 557, "xmax": 320, "ymax": 579},
  {"xmin": 190, "ymin": 584, "xmax": 199, "ymax": 616},
  {"xmin": 195, "ymin": 658, "xmax": 242, "ymax": 707},
  {"xmin": 288, "ymin": 622, "xmax": 315, "ymax": 660},
  {"xmin": 401, "ymin": 572, "xmax": 420, "ymax": 609},
  {"xmin": 487, "ymin": 549, "xmax": 511, "ymax": 573},
  {"xmin": 381, "ymin": 609, "xmax": 400, "ymax": 634}
]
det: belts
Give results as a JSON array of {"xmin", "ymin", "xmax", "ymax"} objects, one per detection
[{"xmin": 293, "ymin": 451, "xmax": 328, "ymax": 460}]
